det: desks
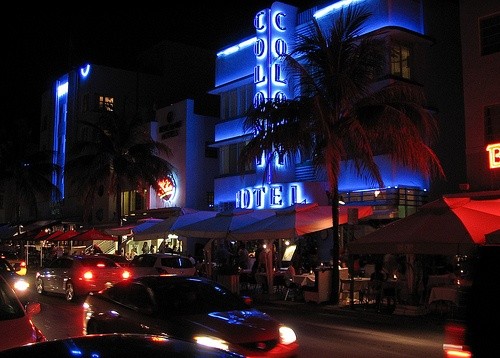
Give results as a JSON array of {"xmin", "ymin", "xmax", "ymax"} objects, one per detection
[
  {"xmin": 429, "ymin": 285, "xmax": 468, "ymax": 317},
  {"xmin": 255, "ymin": 271, "xmax": 268, "ymax": 294},
  {"xmin": 341, "ymin": 277, "xmax": 370, "ymax": 307}
]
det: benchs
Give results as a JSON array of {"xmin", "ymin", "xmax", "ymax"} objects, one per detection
[{"xmin": 302, "ymin": 267, "xmax": 332, "ymax": 304}]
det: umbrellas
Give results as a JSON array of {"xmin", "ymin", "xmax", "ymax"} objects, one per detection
[
  {"xmin": 38, "ymin": 231, "xmax": 63, "ymax": 240},
  {"xmin": 347, "ymin": 195, "xmax": 500, "ymax": 256},
  {"xmin": 175, "ymin": 208, "xmax": 277, "ymax": 238},
  {"xmin": 64, "ymin": 227, "xmax": 118, "ymax": 241},
  {"xmin": 48, "ymin": 229, "xmax": 81, "ymax": 240},
  {"xmin": 135, "ymin": 208, "xmax": 221, "ymax": 241},
  {"xmin": 227, "ymin": 202, "xmax": 374, "ymax": 239},
  {"xmin": 129, "ymin": 217, "xmax": 164, "ymax": 238},
  {"xmin": 9, "ymin": 231, "xmax": 50, "ymax": 240}
]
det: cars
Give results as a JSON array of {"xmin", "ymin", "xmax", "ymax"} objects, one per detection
[
  {"xmin": 130, "ymin": 253, "xmax": 200, "ymax": 278},
  {"xmin": 0, "ymin": 274, "xmax": 47, "ymax": 351},
  {"xmin": 35, "ymin": 254, "xmax": 135, "ymax": 303},
  {"xmin": 93, "ymin": 253, "xmax": 136, "ymax": 280},
  {"xmin": 0, "ymin": 332, "xmax": 246, "ymax": 358},
  {"xmin": 0, "ymin": 245, "xmax": 40, "ymax": 301},
  {"xmin": 83, "ymin": 273, "xmax": 303, "ymax": 358}
]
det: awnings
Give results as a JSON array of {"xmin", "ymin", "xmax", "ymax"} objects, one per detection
[{"xmin": 106, "ymin": 225, "xmax": 135, "ymax": 234}]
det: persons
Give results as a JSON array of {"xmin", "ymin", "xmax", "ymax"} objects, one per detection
[
  {"xmin": 193, "ymin": 239, "xmax": 386, "ymax": 310},
  {"xmin": 0, "ymin": 240, "xmax": 180, "ymax": 268}
]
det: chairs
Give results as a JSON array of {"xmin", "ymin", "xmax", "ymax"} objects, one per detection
[
  {"xmin": 370, "ymin": 281, "xmax": 411, "ymax": 313},
  {"xmin": 238, "ymin": 271, "xmax": 301, "ymax": 302}
]
